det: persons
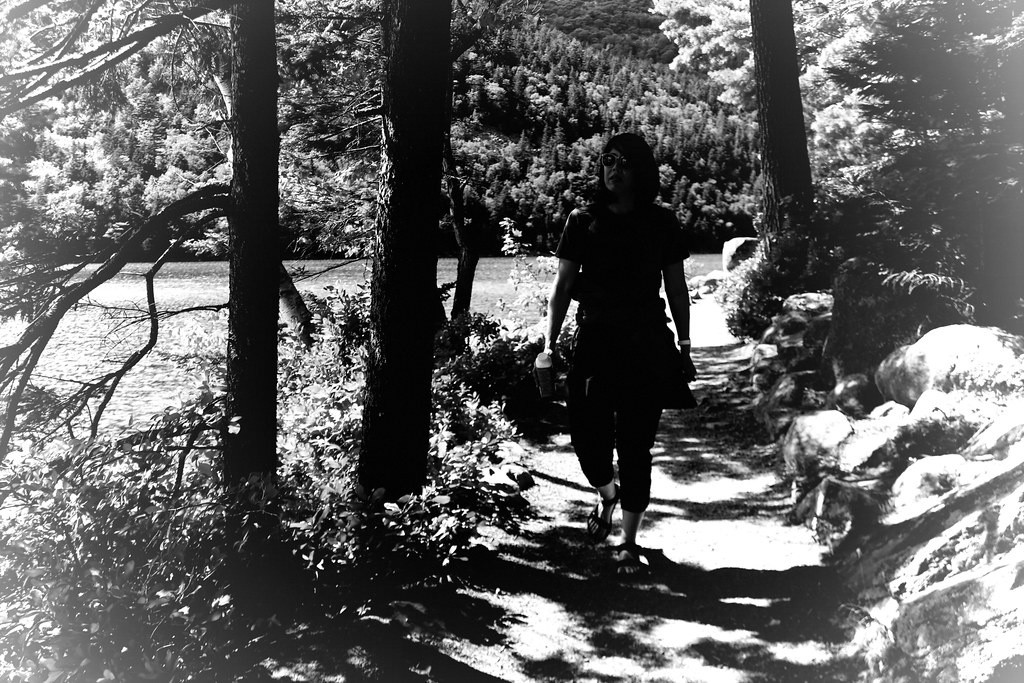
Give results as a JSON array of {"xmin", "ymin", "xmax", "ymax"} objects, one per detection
[{"xmin": 534, "ymin": 133, "xmax": 697, "ymax": 580}]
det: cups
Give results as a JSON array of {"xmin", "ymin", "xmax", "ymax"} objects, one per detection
[{"xmin": 535, "ymin": 363, "xmax": 555, "ymax": 398}]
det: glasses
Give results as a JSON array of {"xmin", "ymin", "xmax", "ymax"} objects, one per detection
[{"xmin": 601, "ymin": 153, "xmax": 636, "ymax": 170}]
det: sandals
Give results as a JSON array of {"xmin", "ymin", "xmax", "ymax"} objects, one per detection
[
  {"xmin": 587, "ymin": 483, "xmax": 620, "ymax": 542},
  {"xmin": 617, "ymin": 543, "xmax": 640, "ymax": 579}
]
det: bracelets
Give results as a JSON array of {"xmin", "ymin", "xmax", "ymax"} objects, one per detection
[{"xmin": 678, "ymin": 340, "xmax": 690, "ymax": 345}]
